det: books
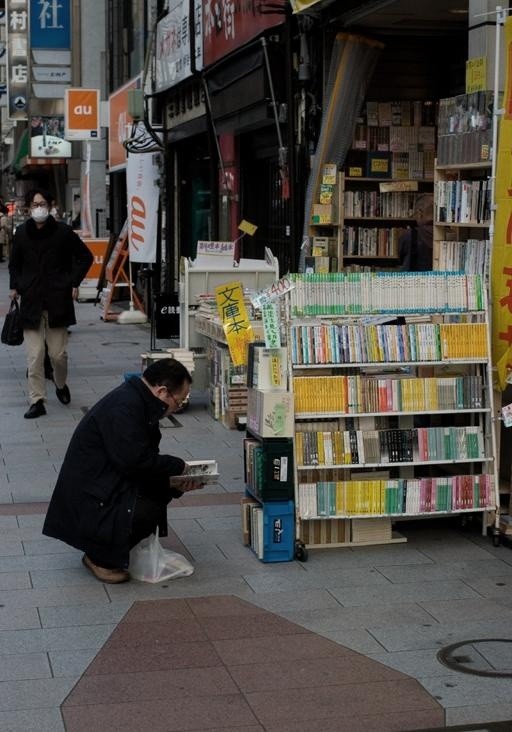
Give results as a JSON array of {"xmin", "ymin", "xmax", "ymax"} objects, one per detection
[
  {"xmin": 141, "ymin": 348, "xmax": 196, "ymax": 376},
  {"xmin": 169, "ymin": 458, "xmax": 218, "ymax": 489},
  {"xmin": 243, "ymin": 270, "xmax": 496, "ymax": 562},
  {"xmin": 196, "ymin": 291, "xmax": 248, "ymax": 430},
  {"xmin": 304, "ymin": 91, "xmax": 500, "ymax": 296}
]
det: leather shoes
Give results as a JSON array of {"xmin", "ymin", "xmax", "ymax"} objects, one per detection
[
  {"xmin": 50, "ymin": 372, "xmax": 71, "ymax": 404},
  {"xmin": 24, "ymin": 399, "xmax": 46, "ymax": 418}
]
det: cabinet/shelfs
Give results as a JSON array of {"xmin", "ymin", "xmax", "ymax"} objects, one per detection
[
  {"xmin": 178, "ymin": 256, "xmax": 279, "ymax": 410},
  {"xmin": 431, "ymin": 158, "xmax": 492, "ymax": 273},
  {"xmin": 285, "ymin": 278, "xmax": 502, "ymax": 562},
  {"xmin": 331, "ymin": 171, "xmax": 433, "ymax": 273}
]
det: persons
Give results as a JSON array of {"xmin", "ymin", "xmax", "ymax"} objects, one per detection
[
  {"xmin": 40, "ymin": 358, "xmax": 192, "ymax": 585},
  {"xmin": 0, "ymin": 192, "xmax": 96, "ymax": 419}
]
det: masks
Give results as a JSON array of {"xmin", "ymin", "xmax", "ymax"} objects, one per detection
[{"xmin": 30, "ymin": 207, "xmax": 50, "ymax": 223}]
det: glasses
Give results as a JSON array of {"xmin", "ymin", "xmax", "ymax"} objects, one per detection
[
  {"xmin": 29, "ymin": 201, "xmax": 49, "ymax": 209},
  {"xmin": 166, "ymin": 389, "xmax": 183, "ymax": 413}
]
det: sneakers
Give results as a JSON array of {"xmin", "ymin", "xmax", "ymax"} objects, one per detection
[{"xmin": 82, "ymin": 552, "xmax": 130, "ymax": 584}]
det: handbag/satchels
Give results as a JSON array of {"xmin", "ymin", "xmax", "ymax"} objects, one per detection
[{"xmin": 1, "ymin": 297, "xmax": 24, "ymax": 345}]
